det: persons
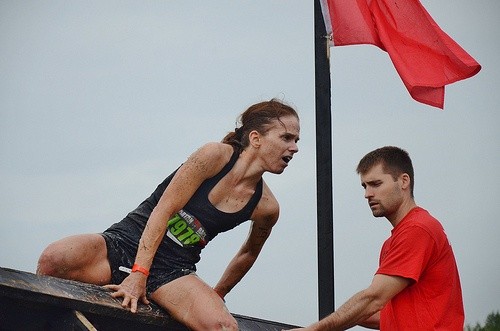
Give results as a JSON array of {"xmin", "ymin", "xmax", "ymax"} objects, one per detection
[
  {"xmin": 288, "ymin": 145, "xmax": 465, "ymax": 331},
  {"xmin": 319, "ymin": 0, "xmax": 483, "ymax": 109},
  {"xmin": 34, "ymin": 99, "xmax": 301, "ymax": 331}
]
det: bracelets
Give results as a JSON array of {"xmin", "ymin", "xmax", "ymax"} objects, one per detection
[
  {"xmin": 213, "ymin": 288, "xmax": 226, "ymax": 303},
  {"xmin": 132, "ymin": 264, "xmax": 149, "ymax": 276}
]
